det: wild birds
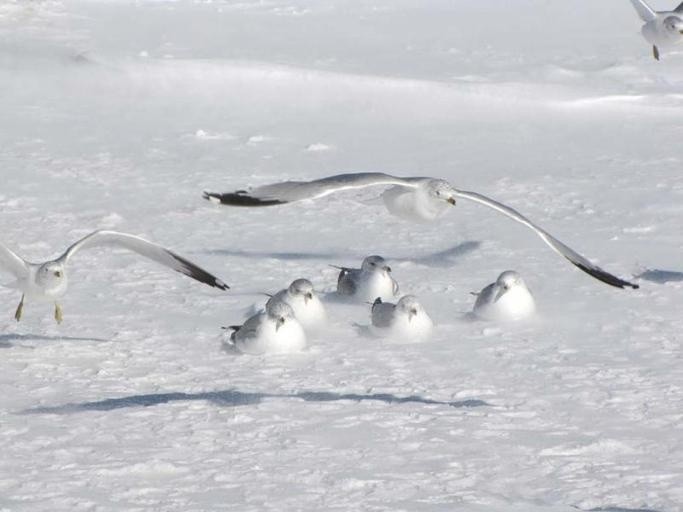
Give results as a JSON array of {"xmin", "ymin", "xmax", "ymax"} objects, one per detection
[
  {"xmin": 202, "ymin": 173, "xmax": 639, "ymax": 289},
  {"xmin": 219, "ymin": 298, "xmax": 306, "ymax": 355},
  {"xmin": 364, "ymin": 296, "xmax": 433, "ymax": 343},
  {"xmin": 328, "ymin": 255, "xmax": 398, "ymax": 303},
  {"xmin": 630, "ymin": 0, "xmax": 683, "ymax": 61},
  {"xmin": 258, "ymin": 279, "xmax": 325, "ymax": 330},
  {"xmin": 0, "ymin": 229, "xmax": 229, "ymax": 325},
  {"xmin": 470, "ymin": 270, "xmax": 535, "ymax": 322}
]
